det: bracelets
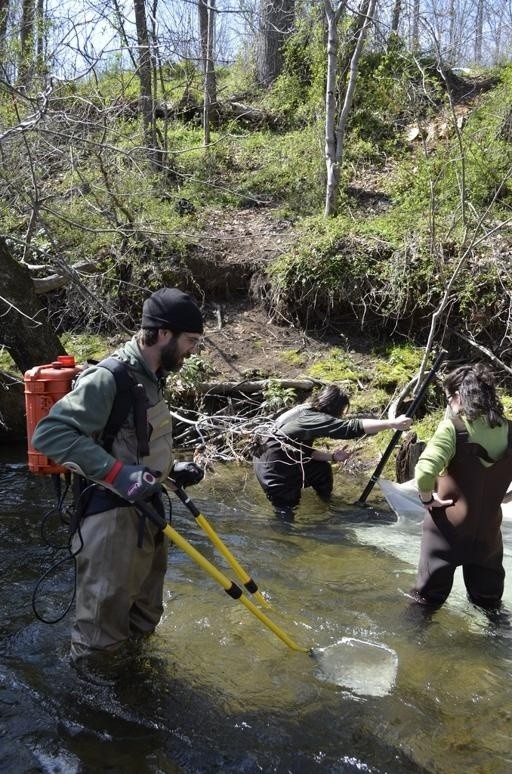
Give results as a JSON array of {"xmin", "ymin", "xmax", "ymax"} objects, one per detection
[{"xmin": 418, "ymin": 493, "xmax": 436, "ymax": 506}]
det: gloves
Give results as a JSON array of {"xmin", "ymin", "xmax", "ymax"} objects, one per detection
[
  {"xmin": 173, "ymin": 456, "xmax": 205, "ymax": 488},
  {"xmin": 114, "ymin": 461, "xmax": 161, "ymax": 502}
]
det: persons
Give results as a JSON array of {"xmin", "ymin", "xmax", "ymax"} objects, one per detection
[
  {"xmin": 401, "ymin": 358, "xmax": 512, "ymax": 644},
  {"xmin": 251, "ymin": 382, "xmax": 415, "ymax": 525},
  {"xmin": 30, "ymin": 286, "xmax": 205, "ymax": 690}
]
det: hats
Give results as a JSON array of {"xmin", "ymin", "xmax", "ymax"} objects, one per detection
[{"xmin": 140, "ymin": 285, "xmax": 206, "ymax": 335}]
